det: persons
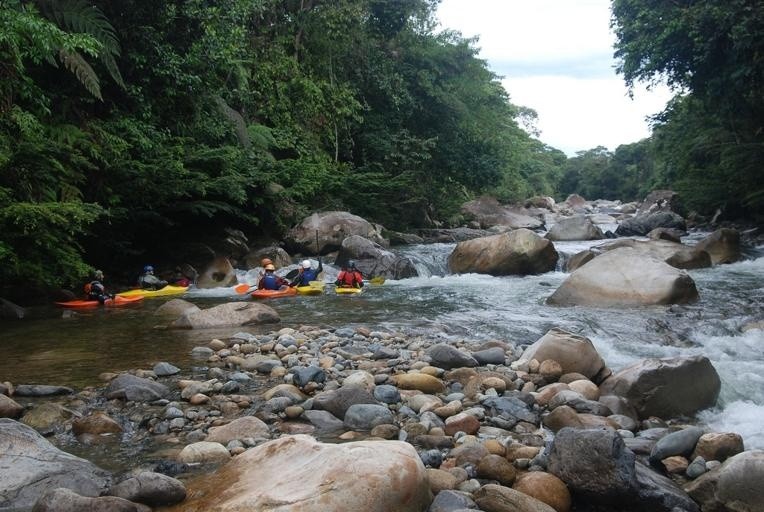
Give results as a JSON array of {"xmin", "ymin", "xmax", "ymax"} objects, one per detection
[
  {"xmin": 334, "ymin": 259, "xmax": 364, "ymax": 289},
  {"xmin": 258, "ymin": 264, "xmax": 288, "ymax": 292},
  {"xmin": 138, "ymin": 266, "xmax": 168, "ymax": 290},
  {"xmin": 286, "ymin": 255, "xmax": 325, "ymax": 287},
  {"xmin": 82, "ymin": 269, "xmax": 116, "ymax": 305},
  {"xmin": 257, "ymin": 258, "xmax": 289, "ymax": 286}
]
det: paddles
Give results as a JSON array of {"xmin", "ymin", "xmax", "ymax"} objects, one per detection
[
  {"xmin": 308, "ymin": 275, "xmax": 385, "ymax": 288},
  {"xmin": 235, "ymin": 276, "xmax": 285, "ymax": 294},
  {"xmin": 247, "ymin": 269, "xmax": 300, "ymax": 295},
  {"xmin": 133, "ymin": 278, "xmax": 190, "ymax": 289}
]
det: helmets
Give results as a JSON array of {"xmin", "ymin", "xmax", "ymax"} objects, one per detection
[
  {"xmin": 302, "ymin": 260, "xmax": 310, "ymax": 269},
  {"xmin": 261, "ymin": 258, "xmax": 272, "ymax": 267},
  {"xmin": 264, "ymin": 264, "xmax": 275, "ymax": 270},
  {"xmin": 94, "ymin": 269, "xmax": 103, "ymax": 279},
  {"xmin": 143, "ymin": 265, "xmax": 154, "ymax": 273}
]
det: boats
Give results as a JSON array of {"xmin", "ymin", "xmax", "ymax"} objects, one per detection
[
  {"xmin": 115, "ymin": 284, "xmax": 190, "ymax": 299},
  {"xmin": 334, "ymin": 285, "xmax": 363, "ymax": 295},
  {"xmin": 294, "ymin": 285, "xmax": 323, "ymax": 295},
  {"xmin": 251, "ymin": 285, "xmax": 298, "ymax": 299},
  {"xmin": 52, "ymin": 295, "xmax": 144, "ymax": 310}
]
